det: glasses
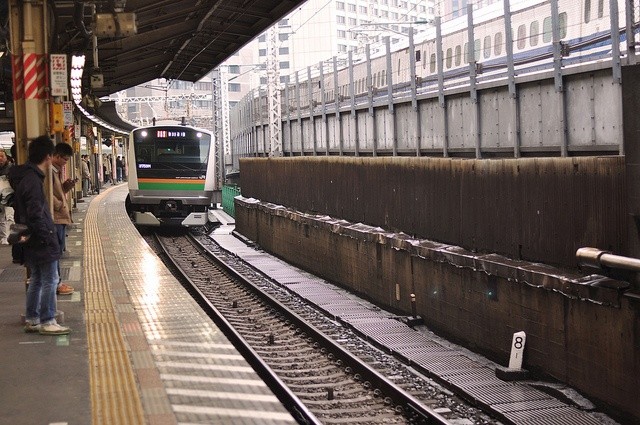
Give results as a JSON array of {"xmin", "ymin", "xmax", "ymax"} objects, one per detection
[{"xmin": 62, "ymin": 156, "xmax": 69, "ymax": 160}]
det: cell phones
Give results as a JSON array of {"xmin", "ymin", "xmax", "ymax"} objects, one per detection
[{"xmin": 67, "ymin": 178, "xmax": 79, "ymax": 183}]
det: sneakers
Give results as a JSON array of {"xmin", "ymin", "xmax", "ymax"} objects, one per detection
[
  {"xmin": 39, "ymin": 322, "xmax": 72, "ymax": 335},
  {"xmin": 23, "ymin": 323, "xmax": 41, "ymax": 332}
]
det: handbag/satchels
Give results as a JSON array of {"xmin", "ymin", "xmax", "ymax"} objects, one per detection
[{"xmin": 7, "ymin": 223, "xmax": 36, "ymax": 247}]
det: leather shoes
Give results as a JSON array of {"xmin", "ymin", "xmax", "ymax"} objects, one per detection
[
  {"xmin": 56, "ymin": 285, "xmax": 74, "ymax": 294},
  {"xmin": 62, "ymin": 283, "xmax": 74, "ymax": 290}
]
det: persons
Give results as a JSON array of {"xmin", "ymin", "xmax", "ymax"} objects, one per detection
[
  {"xmin": 8, "ymin": 135, "xmax": 71, "ymax": 335},
  {"xmin": 0, "ymin": 148, "xmax": 16, "ymax": 245},
  {"xmin": 81, "ymin": 155, "xmax": 90, "ymax": 197},
  {"xmin": 117, "ymin": 156, "xmax": 123, "ymax": 182},
  {"xmin": 103, "ymin": 153, "xmax": 112, "ymax": 183},
  {"xmin": 121, "ymin": 156, "xmax": 125, "ymax": 180},
  {"xmin": 51, "ymin": 142, "xmax": 78, "ymax": 294}
]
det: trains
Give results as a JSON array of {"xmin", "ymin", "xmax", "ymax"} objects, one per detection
[
  {"xmin": 252, "ymin": 0, "xmax": 640, "ymax": 116},
  {"xmin": 128, "ymin": 117, "xmax": 222, "ymax": 218}
]
